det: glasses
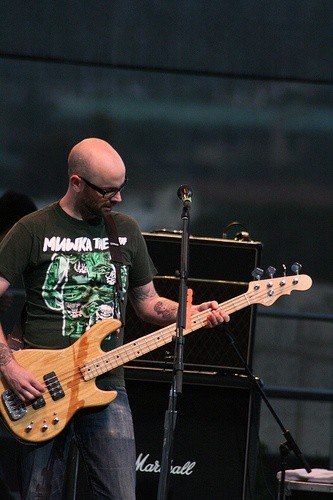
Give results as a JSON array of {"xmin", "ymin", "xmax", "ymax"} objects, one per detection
[{"xmin": 85, "ymin": 178, "xmax": 127, "ymax": 200}]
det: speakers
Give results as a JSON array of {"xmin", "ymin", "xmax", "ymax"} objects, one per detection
[{"xmin": 75, "ymin": 232, "xmax": 265, "ymax": 500}]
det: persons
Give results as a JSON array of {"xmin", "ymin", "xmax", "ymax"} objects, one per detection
[
  {"xmin": 0, "ymin": 189, "xmax": 37, "ymax": 242},
  {"xmin": 0, "ymin": 138, "xmax": 228, "ymax": 499}
]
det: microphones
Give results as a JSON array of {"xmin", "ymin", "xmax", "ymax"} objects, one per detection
[{"xmin": 176, "ymin": 185, "xmax": 193, "ymax": 207}]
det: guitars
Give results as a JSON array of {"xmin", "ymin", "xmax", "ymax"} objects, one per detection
[{"xmin": 0, "ymin": 262, "xmax": 311, "ymax": 445}]
